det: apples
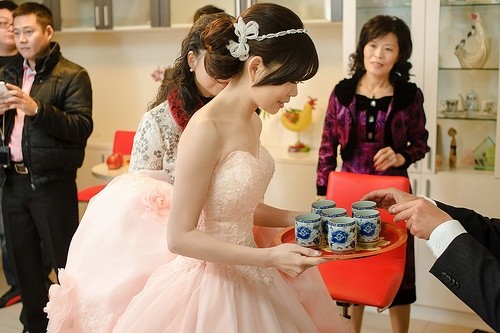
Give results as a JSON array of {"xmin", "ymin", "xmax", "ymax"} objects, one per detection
[{"xmin": 107, "ymin": 154, "xmax": 122, "ymax": 169}]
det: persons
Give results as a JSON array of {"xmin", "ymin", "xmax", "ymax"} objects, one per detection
[
  {"xmin": 361, "ymin": 187, "xmax": 500, "ymax": 333},
  {"xmin": 316, "ymin": 13, "xmax": 429, "ymax": 333},
  {"xmin": 0, "ymin": 1, "xmax": 357, "ymax": 333}
]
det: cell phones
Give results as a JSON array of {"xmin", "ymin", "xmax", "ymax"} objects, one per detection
[{"xmin": 0, "ymin": 80, "xmax": 16, "ymax": 110}]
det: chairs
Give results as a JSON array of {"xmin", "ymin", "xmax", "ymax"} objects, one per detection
[
  {"xmin": 78, "ymin": 129, "xmax": 136, "ymax": 204},
  {"xmin": 280, "ymin": 170, "xmax": 412, "ymax": 333}
]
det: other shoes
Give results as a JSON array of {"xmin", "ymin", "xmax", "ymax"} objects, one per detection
[{"xmin": 0, "ymin": 286, "xmax": 23, "ymax": 308}]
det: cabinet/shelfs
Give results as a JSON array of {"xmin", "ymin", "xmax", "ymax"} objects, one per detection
[
  {"xmin": 53, "ymin": 0, "xmax": 161, "ymax": 33},
  {"xmin": 162, "ymin": 1, "xmax": 344, "ymax": 28},
  {"xmin": 345, "ymin": 0, "xmax": 500, "ymax": 328}
]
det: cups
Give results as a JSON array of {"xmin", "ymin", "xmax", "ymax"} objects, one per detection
[
  {"xmin": 311, "ymin": 200, "xmax": 335, "ymax": 214},
  {"xmin": 327, "ymin": 217, "xmax": 358, "ymax": 252},
  {"xmin": 353, "ymin": 210, "xmax": 381, "ymax": 243},
  {"xmin": 322, "ymin": 207, "xmax": 347, "ymax": 241},
  {"xmin": 294, "ymin": 213, "xmax": 322, "ymax": 249},
  {"xmin": 351, "ymin": 201, "xmax": 378, "ymax": 219}
]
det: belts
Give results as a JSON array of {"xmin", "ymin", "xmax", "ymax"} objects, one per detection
[{"xmin": 11, "ymin": 161, "xmax": 29, "ymax": 175}]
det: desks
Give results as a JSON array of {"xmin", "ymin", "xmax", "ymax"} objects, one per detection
[{"xmin": 92, "ymin": 156, "xmax": 130, "ymax": 181}]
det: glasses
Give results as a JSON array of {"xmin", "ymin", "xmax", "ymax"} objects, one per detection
[{"xmin": 0, "ymin": 22, "xmax": 14, "ymax": 29}]
